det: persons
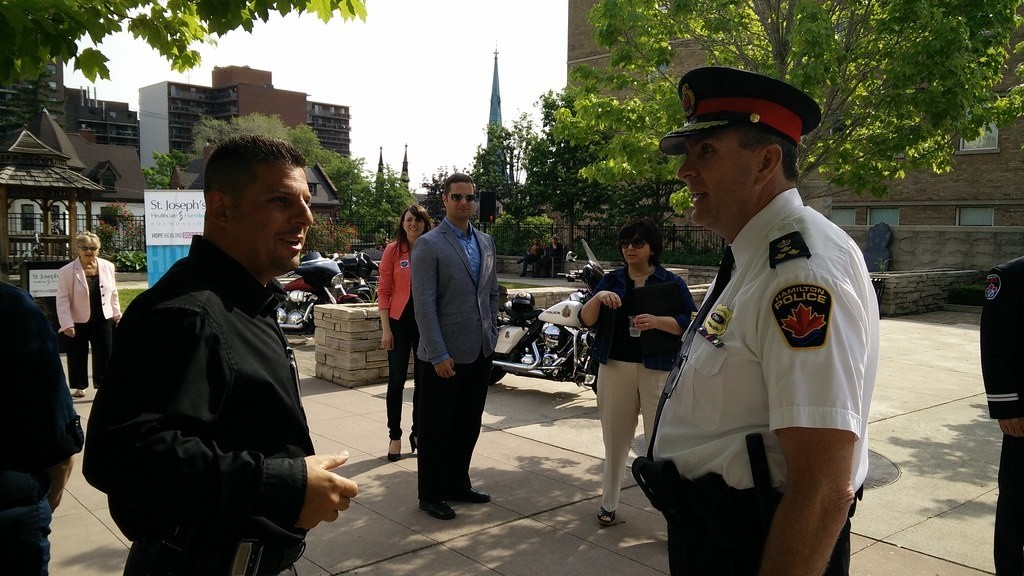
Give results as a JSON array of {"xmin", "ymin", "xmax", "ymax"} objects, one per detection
[
  {"xmin": 516, "ymin": 237, "xmax": 547, "ymax": 277},
  {"xmin": 653, "ymin": 67, "xmax": 880, "ymax": 576},
  {"xmin": 82, "ymin": 133, "xmax": 358, "ymax": 576},
  {"xmin": 577, "ymin": 218, "xmax": 698, "ymax": 523},
  {"xmin": 56, "ymin": 231, "xmax": 121, "ymax": 398},
  {"xmin": 377, "ymin": 204, "xmax": 432, "ymax": 462},
  {"xmin": 0, "ymin": 281, "xmax": 84, "ymax": 576},
  {"xmin": 979, "ymin": 255, "xmax": 1024, "ymax": 575},
  {"xmin": 411, "ymin": 172, "xmax": 499, "ymax": 519},
  {"xmin": 533, "ymin": 237, "xmax": 562, "ymax": 276}
]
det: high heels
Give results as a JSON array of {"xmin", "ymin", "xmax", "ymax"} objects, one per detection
[
  {"xmin": 388, "ymin": 440, "xmax": 401, "ymax": 461},
  {"xmin": 409, "ymin": 433, "xmax": 418, "ymax": 453}
]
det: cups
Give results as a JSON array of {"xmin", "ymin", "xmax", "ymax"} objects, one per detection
[{"xmin": 629, "ymin": 316, "xmax": 641, "ymax": 337}]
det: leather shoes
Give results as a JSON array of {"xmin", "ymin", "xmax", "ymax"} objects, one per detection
[
  {"xmin": 443, "ymin": 488, "xmax": 491, "ymax": 503},
  {"xmin": 419, "ymin": 500, "xmax": 456, "ymax": 520}
]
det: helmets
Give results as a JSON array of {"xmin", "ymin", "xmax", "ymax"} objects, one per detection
[{"xmin": 514, "ymin": 290, "xmax": 535, "ymax": 310}]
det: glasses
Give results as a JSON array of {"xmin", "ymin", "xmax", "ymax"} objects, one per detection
[
  {"xmin": 447, "ymin": 194, "xmax": 476, "ymax": 201},
  {"xmin": 79, "ymin": 246, "xmax": 97, "ymax": 251},
  {"xmin": 620, "ymin": 239, "xmax": 649, "ymax": 249}
]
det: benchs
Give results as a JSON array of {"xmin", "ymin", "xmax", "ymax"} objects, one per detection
[{"xmin": 525, "ymin": 256, "xmax": 565, "ymax": 277}]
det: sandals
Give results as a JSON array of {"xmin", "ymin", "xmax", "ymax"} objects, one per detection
[
  {"xmin": 75, "ymin": 389, "xmax": 86, "ymax": 396},
  {"xmin": 597, "ymin": 505, "xmax": 616, "ymax": 526}
]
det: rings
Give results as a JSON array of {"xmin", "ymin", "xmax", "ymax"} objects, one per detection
[{"xmin": 644, "ymin": 323, "xmax": 649, "ymax": 327}]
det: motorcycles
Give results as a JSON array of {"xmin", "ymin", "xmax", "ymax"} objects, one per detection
[
  {"xmin": 275, "ymin": 250, "xmax": 380, "ymax": 334},
  {"xmin": 487, "ymin": 238, "xmax": 606, "ymax": 395}
]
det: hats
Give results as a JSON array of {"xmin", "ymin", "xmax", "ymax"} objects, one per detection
[{"xmin": 659, "ymin": 67, "xmax": 821, "ymax": 155}]
